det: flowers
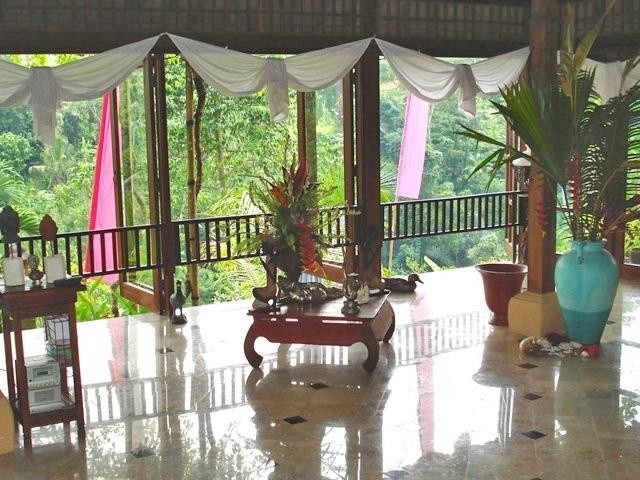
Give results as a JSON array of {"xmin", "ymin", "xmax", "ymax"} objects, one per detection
[{"xmin": 216, "ymin": 134, "xmax": 362, "ymax": 278}]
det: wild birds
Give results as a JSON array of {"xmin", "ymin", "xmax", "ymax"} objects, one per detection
[{"xmin": 169, "ymin": 281, "xmax": 187, "ymax": 324}]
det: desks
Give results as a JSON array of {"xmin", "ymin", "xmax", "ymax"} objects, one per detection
[
  {"xmin": 0, "ymin": 274, "xmax": 87, "ymax": 449},
  {"xmin": 244, "ymin": 289, "xmax": 395, "ymax": 372},
  {"xmin": 245, "ymin": 343, "xmax": 396, "ymax": 426}
]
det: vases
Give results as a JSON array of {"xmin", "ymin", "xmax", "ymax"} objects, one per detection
[
  {"xmin": 472, "ymin": 328, "xmax": 528, "ymax": 388},
  {"xmin": 475, "ymin": 263, "xmax": 528, "ymax": 326}
]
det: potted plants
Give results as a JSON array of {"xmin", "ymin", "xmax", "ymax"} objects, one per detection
[{"xmin": 453, "ymin": 0, "xmax": 640, "ymax": 346}]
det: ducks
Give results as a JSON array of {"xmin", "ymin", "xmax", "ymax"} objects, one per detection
[{"xmin": 382, "ymin": 274, "xmax": 424, "ymax": 293}]
